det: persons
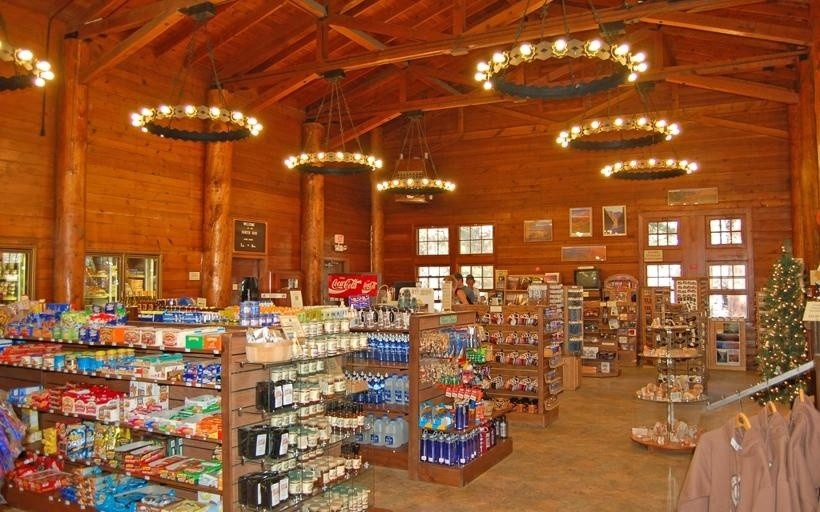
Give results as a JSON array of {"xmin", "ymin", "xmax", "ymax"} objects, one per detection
[
  {"xmin": 452, "ymin": 273, "xmax": 475, "ymax": 305},
  {"xmin": 466, "ymin": 275, "xmax": 480, "ymax": 296},
  {"xmin": 443, "ymin": 275, "xmax": 473, "ymax": 305}
]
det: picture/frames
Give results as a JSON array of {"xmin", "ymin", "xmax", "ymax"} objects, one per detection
[
  {"xmin": 524, "ymin": 204, "xmax": 627, "ymax": 262},
  {"xmin": 233, "ymin": 218, "xmax": 269, "ymax": 255}
]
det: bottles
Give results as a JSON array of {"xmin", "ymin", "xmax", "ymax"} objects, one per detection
[
  {"xmin": 326, "ymin": 403, "xmax": 366, "ymax": 477},
  {"xmin": 419, "ymin": 415, "xmax": 507, "ymax": 465},
  {"xmin": 344, "ymin": 332, "xmax": 410, "ymax": 402}
]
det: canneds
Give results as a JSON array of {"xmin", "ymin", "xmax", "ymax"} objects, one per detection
[{"xmin": 272, "ymin": 318, "xmax": 369, "ymax": 512}]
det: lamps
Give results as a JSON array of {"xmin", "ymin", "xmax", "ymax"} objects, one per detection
[
  {"xmin": 130, "ymin": 2, "xmax": 264, "ymax": 144},
  {"xmin": 473, "ymin": 2, "xmax": 649, "ymax": 97},
  {"xmin": 557, "ymin": 78, "xmax": 680, "ymax": 151},
  {"xmin": 1, "ymin": 2, "xmax": 56, "ymax": 91},
  {"xmin": 600, "ymin": 144, "xmax": 698, "ymax": 182},
  {"xmin": 375, "ymin": 108, "xmax": 456, "ymax": 194},
  {"xmin": 283, "ymin": 69, "xmax": 383, "ymax": 175}
]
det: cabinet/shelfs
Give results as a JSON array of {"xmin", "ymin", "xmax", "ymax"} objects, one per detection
[
  {"xmin": 641, "ymin": 288, "xmax": 746, "ymax": 371},
  {"xmin": 3, "ymin": 286, "xmax": 638, "ymax": 509},
  {"xmin": 630, "ymin": 323, "xmax": 709, "ymax": 454}
]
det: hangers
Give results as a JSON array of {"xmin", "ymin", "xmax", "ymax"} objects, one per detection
[{"xmin": 698, "ymin": 360, "xmax": 819, "ymax": 461}]
